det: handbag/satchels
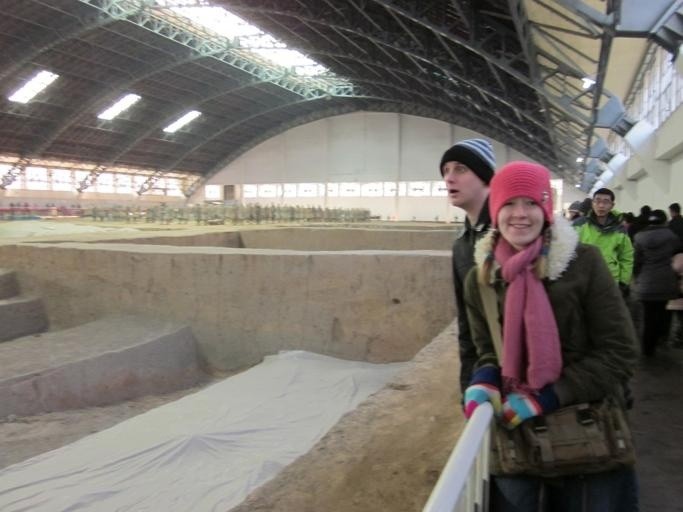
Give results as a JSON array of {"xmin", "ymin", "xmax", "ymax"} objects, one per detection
[{"xmin": 485, "ymin": 390, "xmax": 637, "ymax": 477}]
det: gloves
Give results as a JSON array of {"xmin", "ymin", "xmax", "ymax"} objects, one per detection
[{"xmin": 462, "ymin": 382, "xmax": 545, "ymax": 432}]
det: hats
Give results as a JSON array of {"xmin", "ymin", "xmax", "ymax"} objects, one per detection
[
  {"xmin": 487, "ymin": 160, "xmax": 554, "ymax": 227},
  {"xmin": 438, "ymin": 138, "xmax": 496, "ymax": 184}
]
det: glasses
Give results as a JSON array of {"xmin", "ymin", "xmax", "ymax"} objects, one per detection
[{"xmin": 593, "ymin": 198, "xmax": 612, "ymax": 205}]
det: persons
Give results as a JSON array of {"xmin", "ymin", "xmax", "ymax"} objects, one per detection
[
  {"xmin": 563, "ymin": 186, "xmax": 682, "ymax": 356},
  {"xmin": 438, "ymin": 138, "xmax": 494, "ymax": 411},
  {"xmin": 460, "ymin": 162, "xmax": 641, "ymax": 512}
]
